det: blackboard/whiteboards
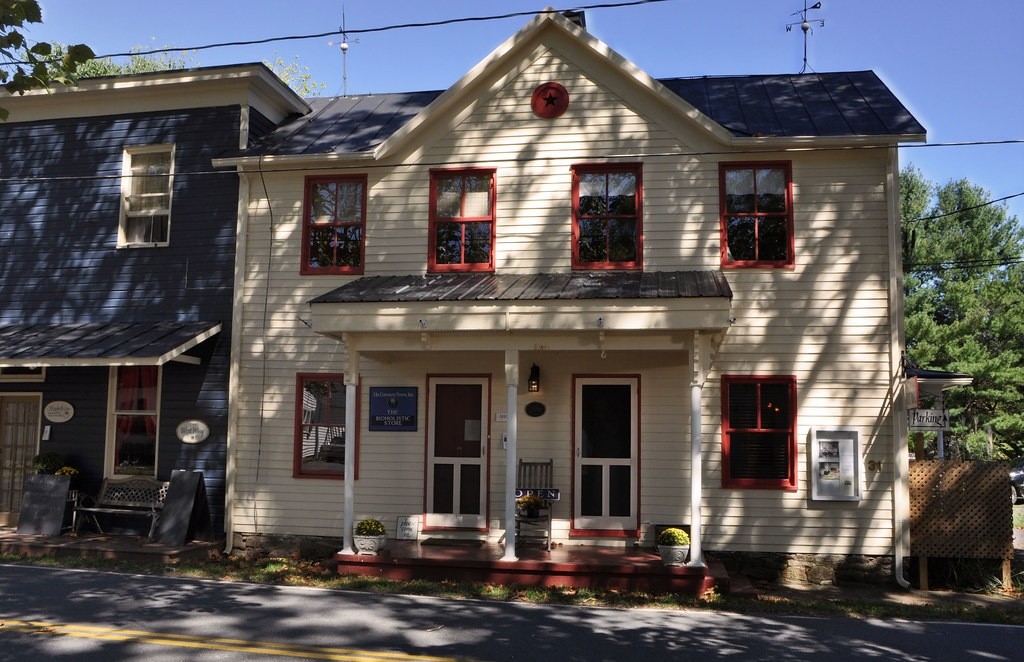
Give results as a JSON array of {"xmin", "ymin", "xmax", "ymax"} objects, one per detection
[
  {"xmin": 153, "ymin": 469, "xmax": 201, "ymax": 546},
  {"xmin": 16, "ymin": 474, "xmax": 71, "ymax": 537}
]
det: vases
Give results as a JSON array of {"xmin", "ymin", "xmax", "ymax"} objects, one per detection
[
  {"xmin": 67, "ymin": 476, "xmax": 77, "ymax": 490},
  {"xmin": 526, "ymin": 509, "xmax": 539, "ymax": 518}
]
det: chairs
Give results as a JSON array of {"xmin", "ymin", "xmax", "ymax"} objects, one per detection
[{"xmin": 515, "ymin": 458, "xmax": 553, "ymax": 551}]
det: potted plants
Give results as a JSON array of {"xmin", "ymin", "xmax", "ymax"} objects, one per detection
[
  {"xmin": 352, "ymin": 519, "xmax": 388, "ymax": 557},
  {"xmin": 655, "ymin": 528, "xmax": 691, "ymax": 566}
]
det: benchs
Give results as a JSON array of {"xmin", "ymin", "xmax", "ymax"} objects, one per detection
[{"xmin": 72, "ymin": 476, "xmax": 170, "ymax": 543}]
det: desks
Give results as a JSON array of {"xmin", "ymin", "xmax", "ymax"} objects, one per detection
[{"xmin": 66, "ymin": 490, "xmax": 79, "ymax": 532}]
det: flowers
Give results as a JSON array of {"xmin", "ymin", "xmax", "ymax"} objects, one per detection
[
  {"xmin": 515, "ymin": 495, "xmax": 545, "ymax": 512},
  {"xmin": 55, "ymin": 466, "xmax": 80, "ymax": 476}
]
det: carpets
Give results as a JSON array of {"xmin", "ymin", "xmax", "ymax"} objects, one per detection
[{"xmin": 421, "ymin": 538, "xmax": 486, "ymax": 548}]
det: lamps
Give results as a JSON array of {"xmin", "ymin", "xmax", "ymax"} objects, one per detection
[{"xmin": 528, "ymin": 365, "xmax": 540, "ymax": 391}]
